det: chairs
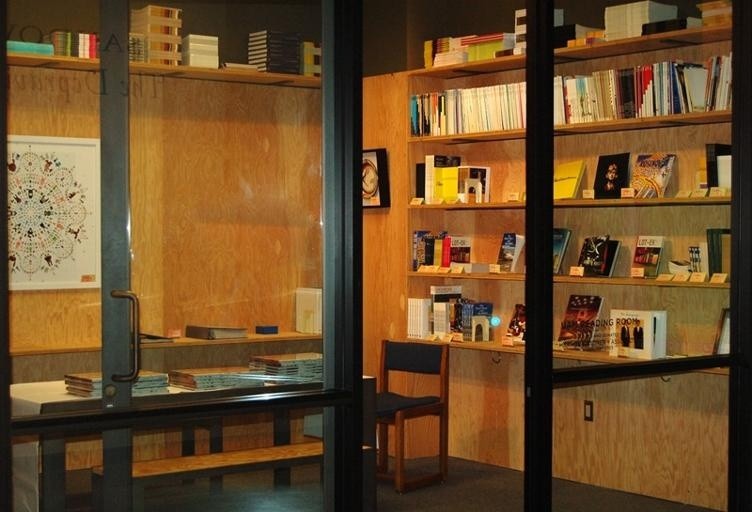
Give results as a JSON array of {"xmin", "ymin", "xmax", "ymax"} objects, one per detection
[{"xmin": 375, "ymin": 339, "xmax": 449, "ymax": 494}]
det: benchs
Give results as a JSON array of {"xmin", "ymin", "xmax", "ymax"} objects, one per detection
[{"xmin": 89, "ymin": 442, "xmax": 375, "ymax": 512}]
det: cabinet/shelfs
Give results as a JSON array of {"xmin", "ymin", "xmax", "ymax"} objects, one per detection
[{"xmin": 406, "ymin": 23, "xmax": 732, "ymax": 376}]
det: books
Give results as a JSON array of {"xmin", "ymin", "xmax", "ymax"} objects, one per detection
[
  {"xmin": 413, "ymin": 230, "xmax": 526, "ymax": 274},
  {"xmin": 416, "ymin": 154, "xmax": 490, "ymax": 203},
  {"xmin": 408, "ymin": 286, "xmax": 526, "ymax": 345},
  {"xmin": 182, "ymin": 30, "xmax": 322, "ymax": 77},
  {"xmin": 62, "ymin": 351, "xmax": 325, "ymax": 399},
  {"xmin": 410, "ymin": 82, "xmax": 526, "ymax": 136},
  {"xmin": 423, "ymin": 7, "xmax": 527, "ymax": 66},
  {"xmin": 554, "ymin": 227, "xmax": 731, "ymax": 282},
  {"xmin": 185, "ymin": 322, "xmax": 248, "ymax": 341},
  {"xmin": 554, "ymin": 144, "xmax": 732, "ymax": 199},
  {"xmin": 5, "ymin": 6, "xmax": 182, "ymax": 67},
  {"xmin": 132, "ymin": 328, "xmax": 176, "ymax": 345},
  {"xmin": 559, "ymin": 293, "xmax": 667, "ymax": 360},
  {"xmin": 553, "ymin": 52, "xmax": 733, "ymax": 126},
  {"xmin": 554, "ymin": 1, "xmax": 734, "ymax": 48}
]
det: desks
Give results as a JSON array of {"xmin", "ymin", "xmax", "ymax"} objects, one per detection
[
  {"xmin": 11, "ymin": 376, "xmax": 378, "ymax": 512},
  {"xmin": 9, "ymin": 331, "xmax": 322, "ymax": 358}
]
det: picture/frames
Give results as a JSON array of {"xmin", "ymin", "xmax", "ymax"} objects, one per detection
[
  {"xmin": 713, "ymin": 308, "xmax": 731, "ymax": 353},
  {"xmin": 362, "ymin": 148, "xmax": 392, "ymax": 208},
  {"xmin": 7, "ymin": 135, "xmax": 101, "ymax": 290}
]
